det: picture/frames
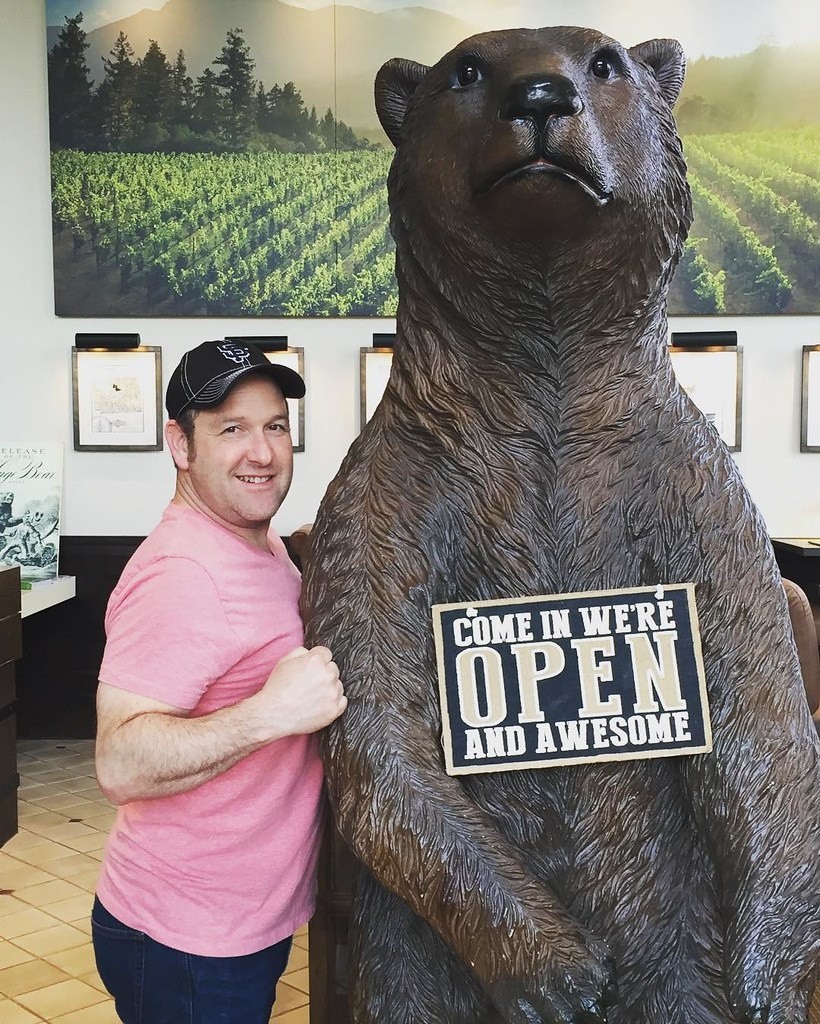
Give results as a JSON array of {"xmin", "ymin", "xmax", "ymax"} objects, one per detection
[
  {"xmin": 361, "ymin": 347, "xmax": 394, "ymax": 432},
  {"xmin": 667, "ymin": 346, "xmax": 743, "ymax": 453},
  {"xmin": 265, "ymin": 347, "xmax": 304, "ymax": 453},
  {"xmin": 801, "ymin": 345, "xmax": 820, "ymax": 453},
  {"xmin": 72, "ymin": 346, "xmax": 163, "ymax": 451}
]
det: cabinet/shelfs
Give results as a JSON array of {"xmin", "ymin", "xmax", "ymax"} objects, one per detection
[{"xmin": 0, "ymin": 565, "xmax": 24, "ymax": 848}]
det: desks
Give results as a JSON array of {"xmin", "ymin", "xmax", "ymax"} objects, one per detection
[
  {"xmin": 22, "ymin": 576, "xmax": 78, "ymax": 618},
  {"xmin": 771, "ymin": 537, "xmax": 820, "ymax": 605}
]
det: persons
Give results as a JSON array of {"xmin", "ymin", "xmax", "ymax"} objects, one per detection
[{"xmin": 92, "ymin": 340, "xmax": 349, "ymax": 1024}]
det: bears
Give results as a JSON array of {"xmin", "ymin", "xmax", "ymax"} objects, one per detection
[{"xmin": 298, "ymin": 26, "xmax": 819, "ymax": 1024}]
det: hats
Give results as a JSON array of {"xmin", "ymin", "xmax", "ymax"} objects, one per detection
[{"xmin": 165, "ymin": 340, "xmax": 306, "ymax": 423}]
